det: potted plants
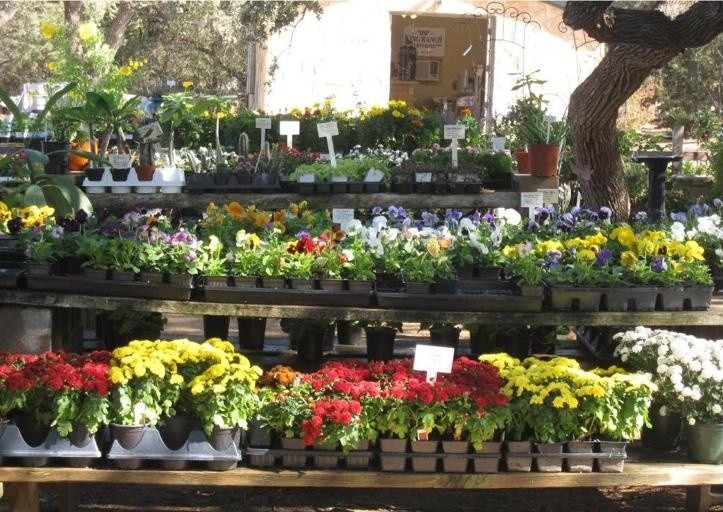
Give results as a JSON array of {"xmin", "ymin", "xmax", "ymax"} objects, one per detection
[
  {"xmin": 418, "ymin": 320, "xmax": 462, "ymax": 350},
  {"xmin": 137, "ymin": 243, "xmax": 166, "ymax": 283},
  {"xmin": 432, "ymin": 166, "xmax": 448, "ymax": 195},
  {"xmin": 295, "ymin": 163, "xmax": 315, "ymax": 194},
  {"xmin": 212, "ymin": 147, "xmax": 230, "ymax": 186},
  {"xmin": 331, "ymin": 166, "xmax": 348, "ymax": 194},
  {"xmin": 84, "ymin": 90, "xmax": 143, "ymax": 182},
  {"xmin": 203, "ymin": 258, "xmax": 230, "ymax": 287},
  {"xmin": 110, "ymin": 238, "xmax": 140, "ymax": 282},
  {"xmin": 280, "ymin": 252, "xmax": 320, "ymax": 290},
  {"xmin": 314, "ymin": 163, "xmax": 331, "ymax": 194},
  {"xmin": 134, "ymin": 126, "xmax": 155, "ymax": 181},
  {"xmin": 346, "ymin": 160, "xmax": 364, "ymax": 193},
  {"xmin": 55, "ymin": 100, "xmax": 105, "ymax": 181},
  {"xmin": 258, "ymin": 250, "xmax": 285, "ymax": 289},
  {"xmin": 353, "ymin": 319, "xmax": 404, "ymax": 362},
  {"xmin": 279, "ymin": 317, "xmax": 328, "ymax": 363},
  {"xmin": 516, "ymin": 259, "xmax": 544, "ymax": 299},
  {"xmin": 95, "ymin": 310, "xmax": 168, "ymax": 351},
  {"xmin": 448, "ymin": 162, "xmax": 489, "ymax": 194},
  {"xmin": 231, "ymin": 252, "xmax": 260, "ymax": 288},
  {"xmin": 234, "ymin": 157, "xmax": 255, "ymax": 186},
  {"xmin": 182, "ymin": 153, "xmax": 212, "ymax": 187},
  {"xmin": 364, "ymin": 158, "xmax": 392, "ymax": 194},
  {"xmin": 317, "ymin": 241, "xmax": 348, "ymax": 291},
  {"xmin": 258, "ymin": 142, "xmax": 283, "ymax": 185},
  {"xmin": 413, "ymin": 161, "xmax": 433, "ymax": 193},
  {"xmin": 508, "ymin": 69, "xmax": 548, "ymax": 174},
  {"xmin": 393, "ymin": 160, "xmax": 413, "ymax": 193},
  {"xmin": 516, "ymin": 99, "xmax": 570, "ymax": 177},
  {"xmin": 374, "ymin": 237, "xmax": 402, "ymax": 293},
  {"xmin": 75, "ymin": 238, "xmax": 114, "ymax": 281},
  {"xmin": 342, "ymin": 235, "xmax": 376, "ymax": 291},
  {"xmin": 30, "ymin": 237, "xmax": 58, "ymax": 277}
]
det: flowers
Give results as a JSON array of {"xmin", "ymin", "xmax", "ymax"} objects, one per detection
[
  {"xmin": 333, "ymin": 380, "xmax": 378, "ymax": 455},
  {"xmin": 547, "ymin": 248, "xmax": 576, "ymax": 284},
  {"xmin": 531, "ymin": 369, "xmax": 578, "ymax": 443},
  {"xmin": 635, "ymin": 239, "xmax": 655, "ymax": 286},
  {"xmin": 608, "ymin": 250, "xmax": 637, "ymax": 288},
  {"xmin": 404, "ymin": 378, "xmax": 447, "ymax": 441},
  {"xmin": 573, "ymin": 375, "xmax": 604, "ymax": 440},
  {"xmin": 684, "ymin": 240, "xmax": 714, "ymax": 286},
  {"xmin": 500, "ymin": 375, "xmax": 536, "ymax": 440},
  {"xmin": 47, "ymin": 362, "xmax": 110, "ymax": 436},
  {"xmin": 468, "ymin": 383, "xmax": 511, "ymax": 450},
  {"xmin": 302, "ymin": 397, "xmax": 361, "ymax": 446},
  {"xmin": 612, "ymin": 325, "xmax": 693, "ymax": 380},
  {"xmin": 485, "ymin": 230, "xmax": 502, "ymax": 266},
  {"xmin": 576, "ymin": 249, "xmax": 611, "ymax": 286},
  {"xmin": 109, "ymin": 352, "xmax": 165, "ymax": 425},
  {"xmin": 601, "ymin": 373, "xmax": 653, "ymax": 442},
  {"xmin": 0, "ymin": 353, "xmax": 45, "ymax": 414},
  {"xmin": 259, "ymin": 389, "xmax": 311, "ymax": 439},
  {"xmin": 655, "ymin": 242, "xmax": 684, "ymax": 286},
  {"xmin": 166, "ymin": 243, "xmax": 199, "ymax": 276},
  {"xmin": 404, "ymin": 239, "xmax": 440, "ymax": 283},
  {"xmin": 162, "ymin": 336, "xmax": 220, "ymax": 419},
  {"xmin": 438, "ymin": 381, "xmax": 472, "ymax": 440},
  {"xmin": 168, "ymin": 273, "xmax": 193, "ymax": 285},
  {"xmin": 657, "ymin": 338, "xmax": 723, "ymax": 426},
  {"xmin": 377, "ymin": 385, "xmax": 414, "ymax": 440},
  {"xmin": 189, "ymin": 363, "xmax": 263, "ymax": 434}
]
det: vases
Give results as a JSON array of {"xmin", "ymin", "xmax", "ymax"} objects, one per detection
[
  {"xmin": 598, "ymin": 440, "xmax": 626, "ymax": 473},
  {"xmin": 411, "ymin": 439, "xmax": 438, "ymax": 473},
  {"xmin": 567, "ymin": 440, "xmax": 594, "ymax": 473},
  {"xmin": 208, "ymin": 428, "xmax": 240, "ymax": 472},
  {"xmin": 659, "ymin": 287, "xmax": 684, "ymax": 311},
  {"xmin": 406, "ymin": 283, "xmax": 429, "ymax": 294},
  {"xmin": 487, "ymin": 267, "xmax": 502, "ymax": 280},
  {"xmin": 578, "ymin": 286, "xmax": 603, "ymax": 312},
  {"xmin": 282, "ymin": 439, "xmax": 307, "ymax": 468},
  {"xmin": 61, "ymin": 421, "xmax": 95, "ymax": 468},
  {"xmin": 472, "ymin": 441, "xmax": 502, "ymax": 474},
  {"xmin": 345, "ymin": 438, "xmax": 369, "ymax": 469},
  {"xmin": 380, "ymin": 439, "xmax": 408, "ymax": 472},
  {"xmin": 686, "ymin": 424, "xmax": 723, "ymax": 464},
  {"xmin": 11, "ymin": 408, "xmax": 50, "ymax": 467},
  {"xmin": 643, "ymin": 405, "xmax": 682, "ymax": 450},
  {"xmin": 605, "ymin": 289, "xmax": 629, "ymax": 311},
  {"xmin": 636, "ymin": 287, "xmax": 657, "ymax": 312},
  {"xmin": 112, "ymin": 424, "xmax": 147, "ymax": 470},
  {"xmin": 314, "ymin": 443, "xmax": 337, "ymax": 467},
  {"xmin": 443, "ymin": 440, "xmax": 469, "ymax": 474},
  {"xmin": 536, "ymin": 440, "xmax": 563, "ymax": 472},
  {"xmin": 163, "ymin": 414, "xmax": 194, "ymax": 471},
  {"xmin": 507, "ymin": 440, "xmax": 533, "ymax": 472},
  {"xmin": 690, "ymin": 287, "xmax": 715, "ymax": 311},
  {"xmin": 550, "ymin": 285, "xmax": 572, "ymax": 313}
]
